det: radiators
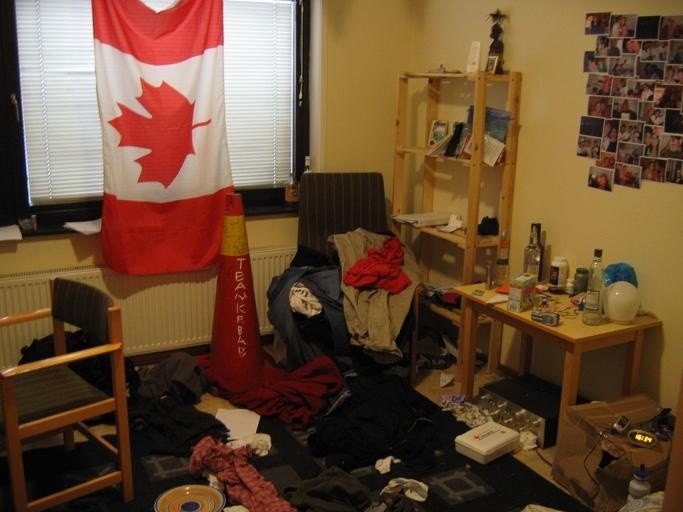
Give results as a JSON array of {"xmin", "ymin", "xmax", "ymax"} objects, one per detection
[{"xmin": 0, "ymin": 244, "xmax": 297, "ymax": 371}]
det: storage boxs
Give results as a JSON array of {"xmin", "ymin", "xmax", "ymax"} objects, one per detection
[{"xmin": 550, "ymin": 393, "xmax": 674, "ymax": 512}]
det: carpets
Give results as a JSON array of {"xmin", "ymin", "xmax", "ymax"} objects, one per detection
[{"xmin": 0, "ymin": 377, "xmax": 591, "ymax": 511}]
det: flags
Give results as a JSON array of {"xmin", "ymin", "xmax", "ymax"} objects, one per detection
[{"xmin": 91, "ymin": 1, "xmax": 235, "ymax": 277}]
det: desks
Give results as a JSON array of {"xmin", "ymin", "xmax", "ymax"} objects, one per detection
[{"xmin": 452, "ymin": 281, "xmax": 661, "ymax": 450}]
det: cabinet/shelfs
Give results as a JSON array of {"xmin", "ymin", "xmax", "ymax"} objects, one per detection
[{"xmin": 388, "ymin": 68, "xmax": 521, "ymax": 378}]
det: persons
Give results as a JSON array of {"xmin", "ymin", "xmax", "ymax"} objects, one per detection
[{"xmin": 574, "ymin": 14, "xmax": 683, "ymax": 192}]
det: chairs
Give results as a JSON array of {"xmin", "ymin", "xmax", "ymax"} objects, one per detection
[
  {"xmin": 0, "ymin": 276, "xmax": 134, "ymax": 511},
  {"xmin": 288, "ymin": 172, "xmax": 416, "ymax": 382}
]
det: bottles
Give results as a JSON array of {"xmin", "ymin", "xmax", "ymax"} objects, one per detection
[
  {"xmin": 582, "ymin": 248, "xmax": 605, "ymax": 326},
  {"xmin": 494, "ymin": 227, "xmax": 511, "ymax": 288},
  {"xmin": 548, "ymin": 254, "xmax": 588, "ymax": 299},
  {"xmin": 522, "ymin": 223, "xmax": 543, "ymax": 282}
]
